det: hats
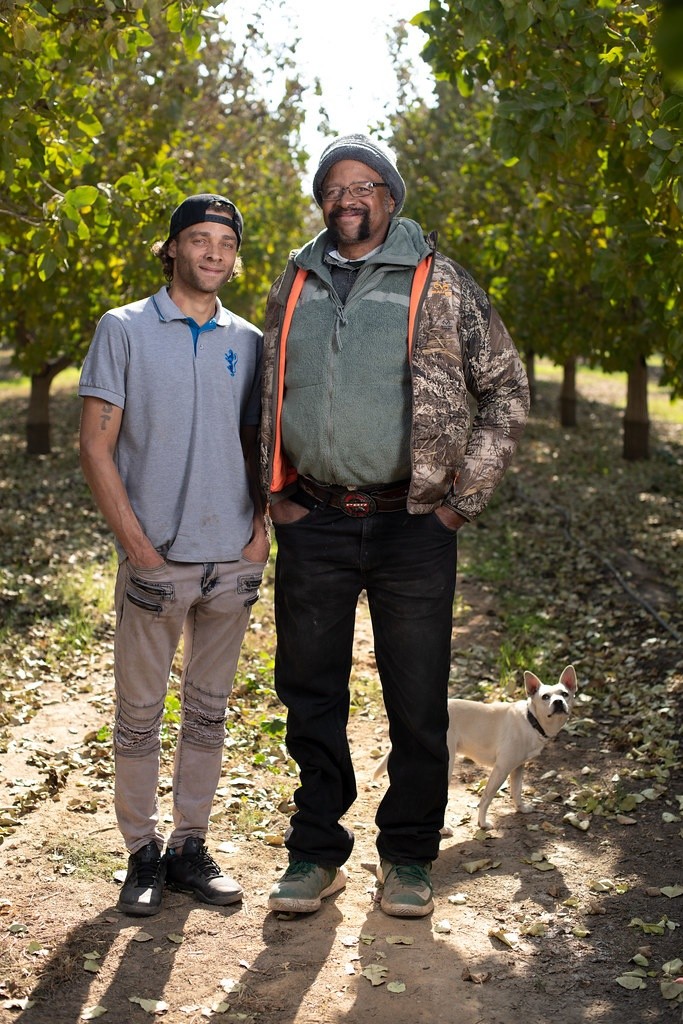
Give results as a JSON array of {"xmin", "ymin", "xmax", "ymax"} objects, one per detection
[
  {"xmin": 161, "ymin": 194, "xmax": 243, "ymax": 251},
  {"xmin": 313, "ymin": 133, "xmax": 406, "ymax": 219}
]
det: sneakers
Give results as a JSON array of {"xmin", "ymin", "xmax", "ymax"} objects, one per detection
[
  {"xmin": 117, "ymin": 841, "xmax": 165, "ymax": 915},
  {"xmin": 267, "ymin": 858, "xmax": 350, "ymax": 913},
  {"xmin": 375, "ymin": 858, "xmax": 434, "ymax": 917},
  {"xmin": 166, "ymin": 837, "xmax": 243, "ymax": 905}
]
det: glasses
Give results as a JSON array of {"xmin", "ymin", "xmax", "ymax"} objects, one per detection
[{"xmin": 318, "ymin": 181, "xmax": 389, "ymax": 200}]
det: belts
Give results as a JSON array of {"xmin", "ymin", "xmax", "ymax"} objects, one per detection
[{"xmin": 296, "ymin": 474, "xmax": 408, "ymax": 516}]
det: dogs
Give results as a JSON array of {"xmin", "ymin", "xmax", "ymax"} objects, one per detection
[{"xmin": 371, "ymin": 664, "xmax": 578, "ymax": 838}]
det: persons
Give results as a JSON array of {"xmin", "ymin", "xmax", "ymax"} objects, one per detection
[
  {"xmin": 79, "ymin": 194, "xmax": 270, "ymax": 915},
  {"xmin": 260, "ymin": 135, "xmax": 530, "ymax": 917}
]
